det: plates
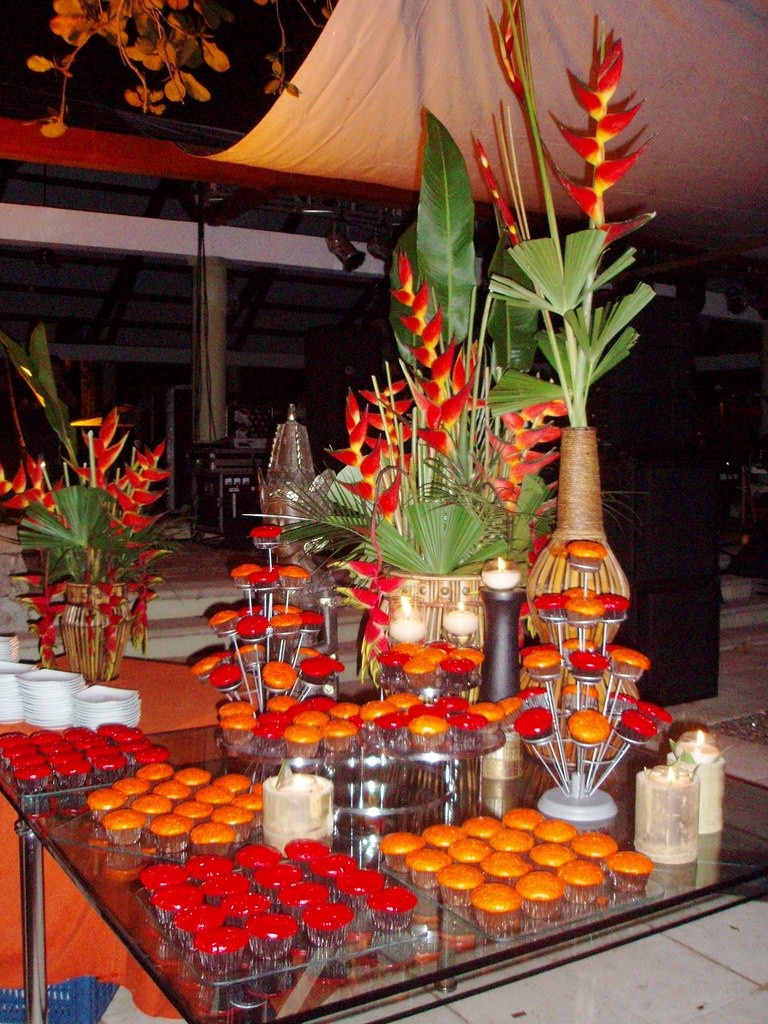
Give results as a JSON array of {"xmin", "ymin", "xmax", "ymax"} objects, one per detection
[
  {"xmin": 0, "ymin": 660, "xmax": 38, "ymax": 724},
  {"xmin": 0, "ymin": 626, "xmax": 21, "ymax": 662},
  {"xmin": 15, "ymin": 668, "xmax": 87, "ymax": 731},
  {"xmin": 71, "ymin": 683, "xmax": 142, "ymax": 733}
]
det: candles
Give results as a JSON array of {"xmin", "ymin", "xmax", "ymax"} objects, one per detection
[
  {"xmin": 443, "ymin": 602, "xmax": 480, "ymax": 636},
  {"xmin": 637, "ymin": 768, "xmax": 698, "ymax": 864},
  {"xmin": 389, "ymin": 594, "xmax": 426, "ymax": 645},
  {"xmin": 480, "ymin": 555, "xmax": 522, "ymax": 592},
  {"xmin": 262, "ymin": 775, "xmax": 333, "ymax": 857},
  {"xmin": 667, "ymin": 740, "xmax": 724, "ymax": 835}
]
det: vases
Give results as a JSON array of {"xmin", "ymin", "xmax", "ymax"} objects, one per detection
[
  {"xmin": 60, "ymin": 582, "xmax": 131, "ymax": 681},
  {"xmin": 525, "ymin": 427, "xmax": 631, "ymax": 649},
  {"xmin": 391, "ymin": 569, "xmax": 489, "ymax": 702}
]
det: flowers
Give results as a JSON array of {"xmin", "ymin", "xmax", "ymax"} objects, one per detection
[
  {"xmin": 324, "ymin": 111, "xmax": 566, "ymax": 690},
  {"xmin": 469, "ymin": 0, "xmax": 654, "ymax": 425},
  {"xmin": 0, "ymin": 320, "xmax": 172, "ymax": 668}
]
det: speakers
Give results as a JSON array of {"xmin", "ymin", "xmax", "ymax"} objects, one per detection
[{"xmin": 603, "ymin": 458, "xmax": 720, "ymax": 706}]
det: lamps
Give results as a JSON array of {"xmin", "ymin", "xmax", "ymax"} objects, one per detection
[{"xmin": 327, "ymin": 219, "xmax": 365, "ymax": 272}]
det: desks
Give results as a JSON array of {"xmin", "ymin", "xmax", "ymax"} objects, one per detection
[{"xmin": 188, "ymin": 445, "xmax": 270, "ymax": 539}]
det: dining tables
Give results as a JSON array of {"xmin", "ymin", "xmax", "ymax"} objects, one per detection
[{"xmin": 1, "ymin": 723, "xmax": 768, "ymax": 1024}]
